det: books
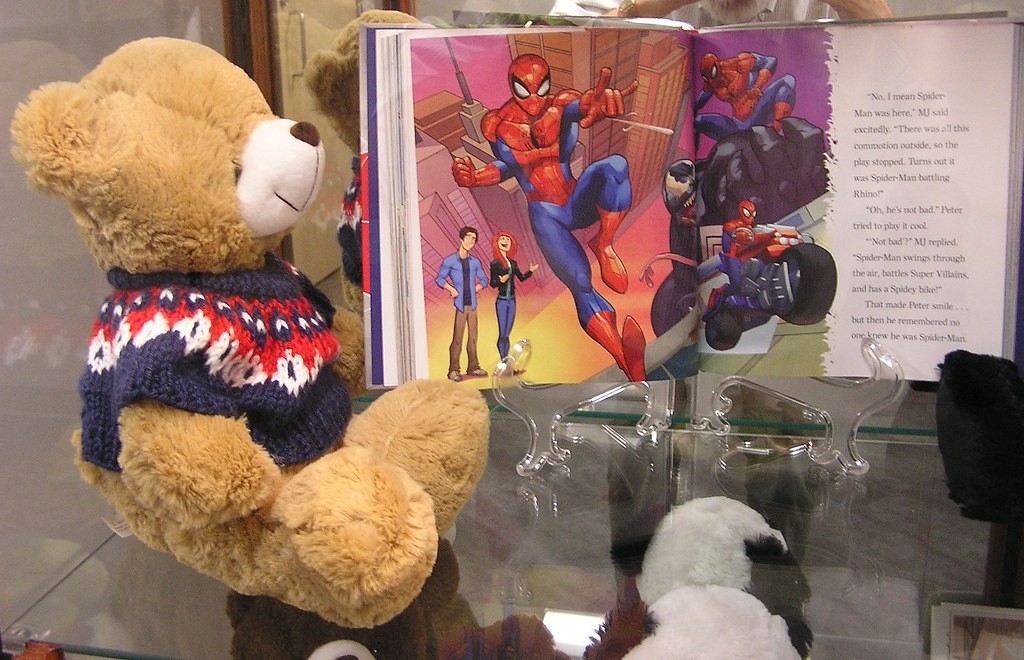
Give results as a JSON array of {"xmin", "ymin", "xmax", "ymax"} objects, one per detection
[{"xmin": 360, "ymin": 12, "xmax": 1024, "ymax": 390}]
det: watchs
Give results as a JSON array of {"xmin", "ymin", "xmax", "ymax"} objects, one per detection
[{"xmin": 618, "ymin": 0, "xmax": 642, "ymax": 18}]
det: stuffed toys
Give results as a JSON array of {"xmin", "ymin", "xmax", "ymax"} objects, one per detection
[
  {"xmin": 304, "ymin": 9, "xmax": 436, "ymax": 312},
  {"xmin": 228, "ymin": 498, "xmax": 811, "ymax": 660},
  {"xmin": 10, "ymin": 38, "xmax": 490, "ymax": 627}
]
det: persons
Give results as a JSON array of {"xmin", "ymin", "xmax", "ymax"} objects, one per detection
[{"xmin": 547, "ymin": 0, "xmax": 892, "ymax": 611}]
